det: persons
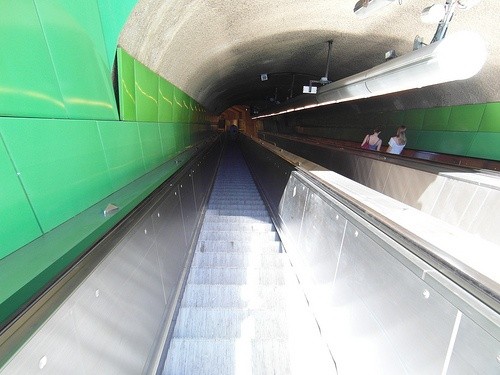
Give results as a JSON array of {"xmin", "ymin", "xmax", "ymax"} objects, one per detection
[
  {"xmin": 360, "ymin": 127, "xmax": 383, "ymax": 152},
  {"xmin": 384, "ymin": 125, "xmax": 408, "ymax": 155}
]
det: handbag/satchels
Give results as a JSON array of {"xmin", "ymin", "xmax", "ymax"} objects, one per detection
[{"xmin": 362, "ymin": 134, "xmax": 370, "ymax": 149}]
